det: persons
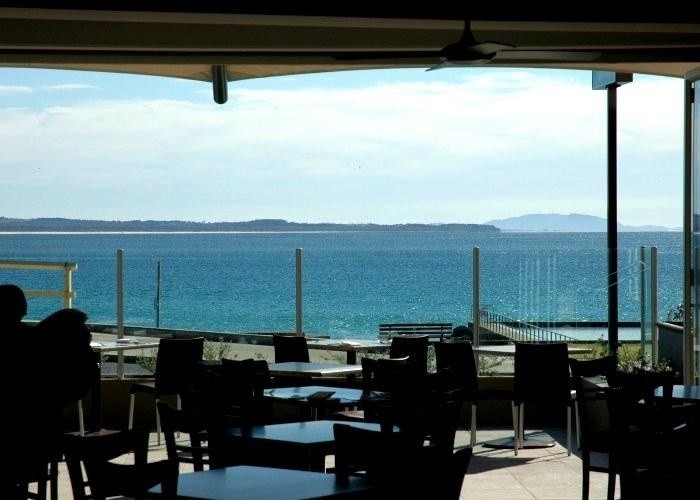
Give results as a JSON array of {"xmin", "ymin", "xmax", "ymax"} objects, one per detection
[{"xmin": 2, "ymin": 285, "xmax": 30, "ymax": 372}]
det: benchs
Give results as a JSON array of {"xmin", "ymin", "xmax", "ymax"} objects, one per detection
[{"xmin": 376, "ymin": 322, "xmax": 455, "ymax": 343}]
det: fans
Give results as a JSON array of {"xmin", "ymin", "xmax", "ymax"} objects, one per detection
[{"xmin": 334, "ymin": 0, "xmax": 518, "ymax": 73}]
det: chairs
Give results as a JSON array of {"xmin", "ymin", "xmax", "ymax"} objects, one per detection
[
  {"xmin": 568, "ymin": 357, "xmax": 700, "ymax": 500},
  {"xmin": 2, "ymin": 330, "xmax": 472, "ymax": 500},
  {"xmin": 432, "ymin": 339, "xmax": 574, "ymax": 456}
]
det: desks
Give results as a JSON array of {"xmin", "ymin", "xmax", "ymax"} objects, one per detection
[
  {"xmin": 473, "ymin": 345, "xmax": 592, "ymax": 449},
  {"xmin": 584, "ymin": 371, "xmax": 700, "ymax": 500}
]
